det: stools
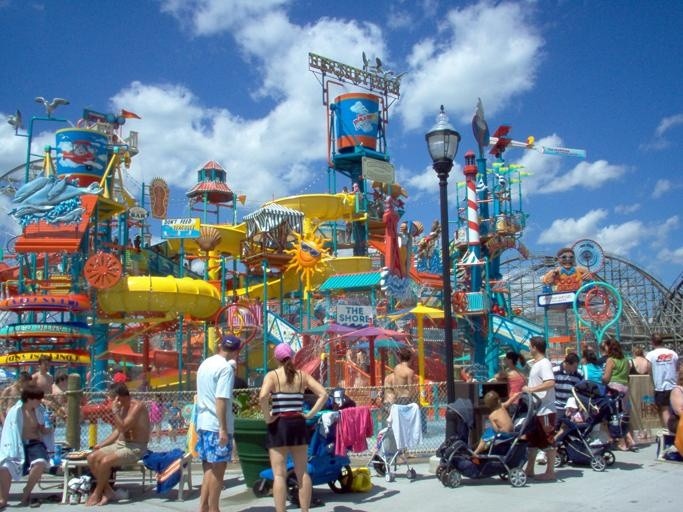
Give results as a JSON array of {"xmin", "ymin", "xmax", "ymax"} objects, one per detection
[{"xmin": 657, "ymin": 429, "xmax": 676, "ymax": 461}]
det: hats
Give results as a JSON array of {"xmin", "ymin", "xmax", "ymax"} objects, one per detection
[
  {"xmin": 222, "ymin": 335, "xmax": 241, "ymax": 350},
  {"xmin": 274, "ymin": 343, "xmax": 292, "ymax": 361},
  {"xmin": 228, "ymin": 359, "xmax": 237, "ymax": 369},
  {"xmin": 564, "ymin": 397, "xmax": 578, "ymax": 408}
]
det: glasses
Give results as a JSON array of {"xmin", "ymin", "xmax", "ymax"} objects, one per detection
[
  {"xmin": 42, "ymin": 363, "xmax": 48, "ymax": 366},
  {"xmin": 504, "ymin": 357, "xmax": 508, "ymax": 360}
]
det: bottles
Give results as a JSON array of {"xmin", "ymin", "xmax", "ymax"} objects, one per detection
[
  {"xmin": 53, "ymin": 444, "xmax": 62, "ymax": 466},
  {"xmin": 43, "ymin": 408, "xmax": 52, "ymax": 429}
]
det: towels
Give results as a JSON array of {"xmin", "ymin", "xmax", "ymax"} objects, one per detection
[
  {"xmin": 0, "ymin": 399, "xmax": 54, "ymax": 481},
  {"xmin": 143, "ymin": 448, "xmax": 188, "ymax": 493}
]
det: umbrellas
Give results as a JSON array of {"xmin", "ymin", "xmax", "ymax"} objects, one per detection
[
  {"xmin": 377, "ymin": 302, "xmax": 463, "ymax": 406},
  {"xmin": 323, "ymin": 326, "xmax": 419, "ymax": 386},
  {"xmin": 351, "ymin": 338, "xmax": 406, "ymax": 386},
  {"xmin": 302, "ymin": 321, "xmax": 356, "ymax": 386}
]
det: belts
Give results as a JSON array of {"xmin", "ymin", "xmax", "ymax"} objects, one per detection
[{"xmin": 23, "ymin": 440, "xmax": 40, "ymax": 444}]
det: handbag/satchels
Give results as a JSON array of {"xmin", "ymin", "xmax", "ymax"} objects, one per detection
[
  {"xmin": 608, "ymin": 411, "xmax": 630, "ymax": 438},
  {"xmin": 342, "ymin": 468, "xmax": 373, "ymax": 491}
]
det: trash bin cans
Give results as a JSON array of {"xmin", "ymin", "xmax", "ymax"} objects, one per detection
[{"xmin": 233, "ymin": 419, "xmax": 273, "ymax": 487}]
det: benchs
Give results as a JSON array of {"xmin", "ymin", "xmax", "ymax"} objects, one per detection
[
  {"xmin": 11, "ymin": 458, "xmax": 76, "ymax": 503},
  {"xmin": 62, "ymin": 449, "xmax": 193, "ymax": 503}
]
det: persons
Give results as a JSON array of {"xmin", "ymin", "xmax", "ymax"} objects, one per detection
[
  {"xmin": 350, "ymin": 183, "xmax": 363, "ymax": 200},
  {"xmin": 578, "ymin": 349, "xmax": 606, "ymax": 397},
  {"xmin": 646, "ymin": 333, "xmax": 678, "ymax": 428},
  {"xmin": 196, "ymin": 335, "xmax": 241, "ymax": 511},
  {"xmin": 113, "ymin": 368, "xmax": 130, "ymax": 384},
  {"xmin": 31, "ymin": 355, "xmax": 53, "ymax": 409},
  {"xmin": 384, "ymin": 373, "xmax": 397, "ymax": 414},
  {"xmin": 543, "ymin": 250, "xmax": 595, "ymax": 295},
  {"xmin": 0, "ymin": 372, "xmax": 56, "ymax": 428},
  {"xmin": 668, "ymin": 357, "xmax": 683, "ymax": 432},
  {"xmin": 134, "ymin": 235, "xmax": 140, "ymax": 250},
  {"xmin": 228, "ymin": 359, "xmax": 247, "ymax": 389},
  {"xmin": 342, "ymin": 187, "xmax": 349, "ymax": 194},
  {"xmin": 357, "ymin": 347, "xmax": 368, "ymax": 374},
  {"xmin": 346, "ymin": 348, "xmax": 358, "ymax": 378},
  {"xmin": 394, "ymin": 349, "xmax": 414, "ymax": 405},
  {"xmin": 474, "ymin": 391, "xmax": 521, "ymax": 454},
  {"xmin": 52, "ymin": 371, "xmax": 68, "ymax": 405},
  {"xmin": 145, "ymin": 395, "xmax": 164, "ymax": 443},
  {"xmin": 259, "ymin": 342, "xmax": 330, "ymax": 512},
  {"xmin": 137, "ymin": 367, "xmax": 160, "ymax": 392},
  {"xmin": 602, "ymin": 340, "xmax": 638, "ymax": 452},
  {"xmin": 167, "ymin": 399, "xmax": 181, "ymax": 442},
  {"xmin": 522, "ymin": 336, "xmax": 556, "ymax": 480},
  {"xmin": 598, "ymin": 341, "xmax": 610, "ymax": 370},
  {"xmin": 0, "ymin": 384, "xmax": 54, "ymax": 508},
  {"xmin": 84, "ymin": 381, "xmax": 150, "ymax": 506},
  {"xmin": 495, "ymin": 352, "xmax": 526, "ymax": 415},
  {"xmin": 628, "ymin": 347, "xmax": 652, "ymax": 375},
  {"xmin": 553, "ymin": 354, "xmax": 585, "ymax": 430},
  {"xmin": 554, "ymin": 397, "xmax": 585, "ymax": 441}
]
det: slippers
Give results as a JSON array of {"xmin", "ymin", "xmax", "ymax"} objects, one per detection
[
  {"xmin": 29, "ymin": 498, "xmax": 40, "ymax": 507},
  {"xmin": 39, "ymin": 494, "xmax": 61, "ymax": 503},
  {"xmin": 616, "ymin": 441, "xmax": 629, "ymax": 451},
  {"xmin": 627, "ymin": 446, "xmax": 639, "ymax": 450}
]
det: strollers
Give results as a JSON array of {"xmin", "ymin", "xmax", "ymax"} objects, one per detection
[
  {"xmin": 555, "ymin": 381, "xmax": 625, "ymax": 471},
  {"xmin": 253, "ymin": 411, "xmax": 353, "ymax": 505},
  {"xmin": 367, "ymin": 404, "xmax": 418, "ymax": 483},
  {"xmin": 435, "ymin": 389, "xmax": 542, "ymax": 488}
]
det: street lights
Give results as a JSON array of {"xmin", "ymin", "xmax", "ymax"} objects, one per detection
[{"xmin": 424, "ymin": 104, "xmax": 461, "ymax": 472}]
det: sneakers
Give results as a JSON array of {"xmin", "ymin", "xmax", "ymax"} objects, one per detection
[
  {"xmin": 70, "ymin": 495, "xmax": 78, "ymax": 504},
  {"xmin": 68, "ymin": 484, "xmax": 81, "ymax": 494},
  {"xmin": 114, "ymin": 487, "xmax": 129, "ymax": 498},
  {"xmin": 80, "ymin": 494, "xmax": 89, "ymax": 504},
  {"xmin": 77, "ymin": 482, "xmax": 92, "ymax": 494}
]
